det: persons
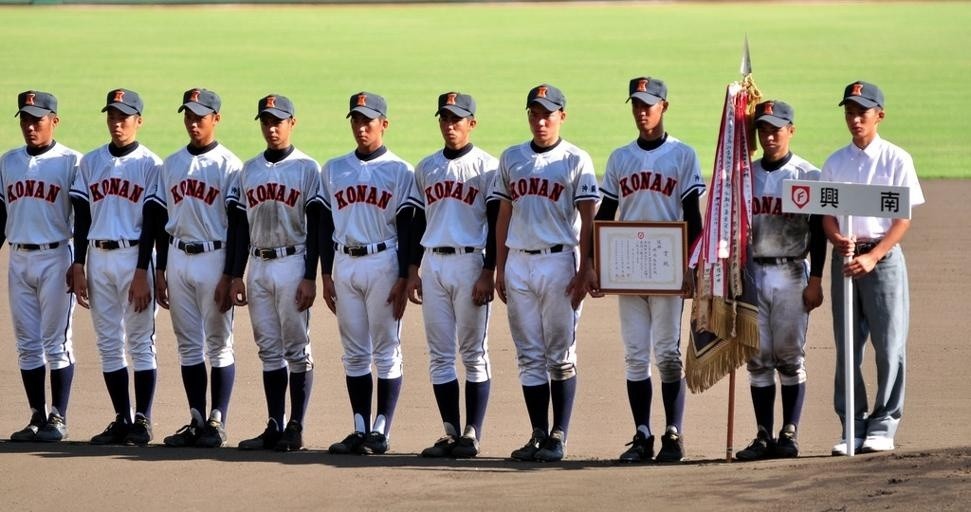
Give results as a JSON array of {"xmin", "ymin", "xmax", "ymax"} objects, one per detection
[
  {"xmin": 68, "ymin": 90, "xmax": 166, "ymax": 447},
  {"xmin": 0, "ymin": 91, "xmax": 83, "ymax": 441},
  {"xmin": 406, "ymin": 92, "xmax": 501, "ymax": 460},
  {"xmin": 494, "ymin": 84, "xmax": 600, "ymax": 463},
  {"xmin": 223, "ymin": 92, "xmax": 320, "ymax": 451},
  {"xmin": 315, "ymin": 92, "xmax": 420, "ymax": 456},
  {"xmin": 815, "ymin": 80, "xmax": 926, "ymax": 456},
  {"xmin": 735, "ymin": 97, "xmax": 827, "ymax": 459},
  {"xmin": 586, "ymin": 75, "xmax": 703, "ymax": 462},
  {"xmin": 159, "ymin": 89, "xmax": 244, "ymax": 449}
]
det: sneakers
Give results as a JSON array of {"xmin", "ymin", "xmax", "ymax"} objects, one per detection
[
  {"xmin": 620, "ymin": 430, "xmax": 655, "ymax": 460},
  {"xmin": 35, "ymin": 413, "xmax": 66, "ymax": 442},
  {"xmin": 534, "ymin": 430, "xmax": 568, "ymax": 462},
  {"xmin": 421, "ymin": 433, "xmax": 460, "ymax": 457},
  {"xmin": 770, "ymin": 431, "xmax": 799, "ymax": 458},
  {"xmin": 126, "ymin": 414, "xmax": 153, "ymax": 444},
  {"xmin": 163, "ymin": 417, "xmax": 205, "ymax": 447},
  {"xmin": 11, "ymin": 414, "xmax": 49, "ymax": 441},
  {"xmin": 510, "ymin": 429, "xmax": 550, "ymax": 462},
  {"xmin": 736, "ymin": 430, "xmax": 777, "ymax": 461},
  {"xmin": 354, "ymin": 431, "xmax": 391, "ymax": 456},
  {"xmin": 191, "ymin": 421, "xmax": 228, "ymax": 448},
  {"xmin": 238, "ymin": 420, "xmax": 284, "ymax": 450},
  {"xmin": 449, "ymin": 434, "xmax": 480, "ymax": 457},
  {"xmin": 832, "ymin": 437, "xmax": 866, "ymax": 456},
  {"xmin": 329, "ymin": 431, "xmax": 366, "ymax": 454},
  {"xmin": 652, "ymin": 430, "xmax": 686, "ymax": 462},
  {"xmin": 860, "ymin": 434, "xmax": 895, "ymax": 451},
  {"xmin": 90, "ymin": 412, "xmax": 132, "ymax": 445},
  {"xmin": 273, "ymin": 424, "xmax": 301, "ymax": 452}
]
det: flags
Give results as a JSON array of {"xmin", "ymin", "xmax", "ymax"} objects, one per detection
[{"xmin": 686, "ymin": 75, "xmax": 763, "ymax": 394}]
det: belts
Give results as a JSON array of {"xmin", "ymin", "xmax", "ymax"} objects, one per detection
[
  {"xmin": 854, "ymin": 243, "xmax": 878, "ymax": 255},
  {"xmin": 8, "ymin": 242, "xmax": 58, "ymax": 249},
  {"xmin": 248, "ymin": 246, "xmax": 295, "ymax": 260},
  {"xmin": 87, "ymin": 240, "xmax": 137, "ymax": 248},
  {"xmin": 335, "ymin": 242, "xmax": 385, "ymax": 256},
  {"xmin": 169, "ymin": 237, "xmax": 220, "ymax": 255},
  {"xmin": 524, "ymin": 244, "xmax": 561, "ymax": 253},
  {"xmin": 432, "ymin": 248, "xmax": 473, "ymax": 253},
  {"xmin": 754, "ymin": 257, "xmax": 794, "ymax": 263}
]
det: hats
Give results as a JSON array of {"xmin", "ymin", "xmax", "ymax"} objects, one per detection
[
  {"xmin": 625, "ymin": 77, "xmax": 667, "ymax": 105},
  {"xmin": 254, "ymin": 94, "xmax": 293, "ymax": 121},
  {"xmin": 435, "ymin": 92, "xmax": 476, "ymax": 118},
  {"xmin": 177, "ymin": 88, "xmax": 221, "ymax": 117},
  {"xmin": 525, "ymin": 85, "xmax": 564, "ymax": 112},
  {"xmin": 346, "ymin": 91, "xmax": 386, "ymax": 119},
  {"xmin": 13, "ymin": 90, "xmax": 57, "ymax": 118},
  {"xmin": 838, "ymin": 81, "xmax": 884, "ymax": 110},
  {"xmin": 101, "ymin": 88, "xmax": 142, "ymax": 116},
  {"xmin": 753, "ymin": 101, "xmax": 793, "ymax": 128}
]
